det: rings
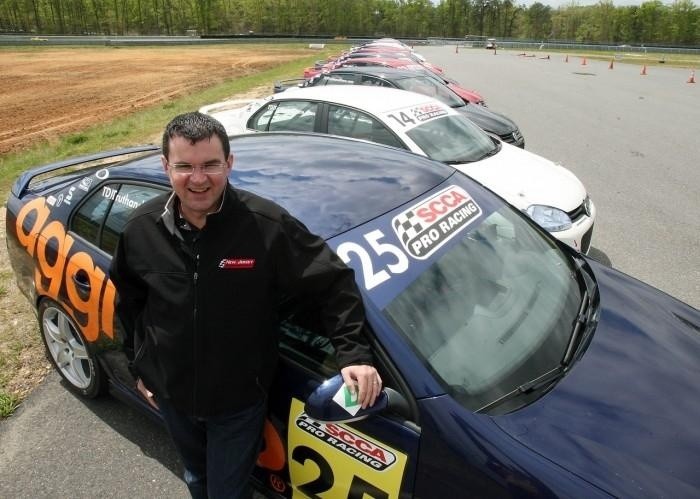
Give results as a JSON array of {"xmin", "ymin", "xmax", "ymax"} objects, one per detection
[{"xmin": 372, "ymin": 381, "xmax": 379, "ymax": 385}]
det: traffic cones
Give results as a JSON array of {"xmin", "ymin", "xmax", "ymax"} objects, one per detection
[
  {"xmin": 566, "ymin": 54, "xmax": 569, "ymax": 62},
  {"xmin": 640, "ymin": 64, "xmax": 647, "ymax": 75},
  {"xmin": 608, "ymin": 59, "xmax": 614, "ymax": 69},
  {"xmin": 581, "ymin": 57, "xmax": 586, "ymax": 65},
  {"xmin": 540, "ymin": 55, "xmax": 550, "ymax": 60},
  {"xmin": 411, "ymin": 41, "xmax": 414, "ymax": 49},
  {"xmin": 518, "ymin": 52, "xmax": 526, "ymax": 56},
  {"xmin": 494, "ymin": 44, "xmax": 498, "ymax": 55},
  {"xmin": 455, "ymin": 43, "xmax": 460, "ymax": 54},
  {"xmin": 526, "ymin": 54, "xmax": 535, "ymax": 57},
  {"xmin": 687, "ymin": 69, "xmax": 696, "ymax": 83}
]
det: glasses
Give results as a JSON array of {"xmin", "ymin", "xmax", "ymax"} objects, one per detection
[{"xmin": 163, "ymin": 160, "xmax": 228, "ymax": 175}]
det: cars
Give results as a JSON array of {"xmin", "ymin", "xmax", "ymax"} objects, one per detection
[
  {"xmin": 272, "ymin": 38, "xmax": 527, "ymax": 150},
  {"xmin": 31, "ymin": 37, "xmax": 49, "ymax": 41},
  {"xmin": 334, "ymin": 35, "xmax": 349, "ymax": 40},
  {"xmin": 197, "ymin": 84, "xmax": 597, "ymax": 257},
  {"xmin": 4, "ymin": 131, "xmax": 700, "ymax": 499}
]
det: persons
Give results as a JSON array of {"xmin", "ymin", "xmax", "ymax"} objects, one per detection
[{"xmin": 109, "ymin": 111, "xmax": 384, "ymax": 499}]
